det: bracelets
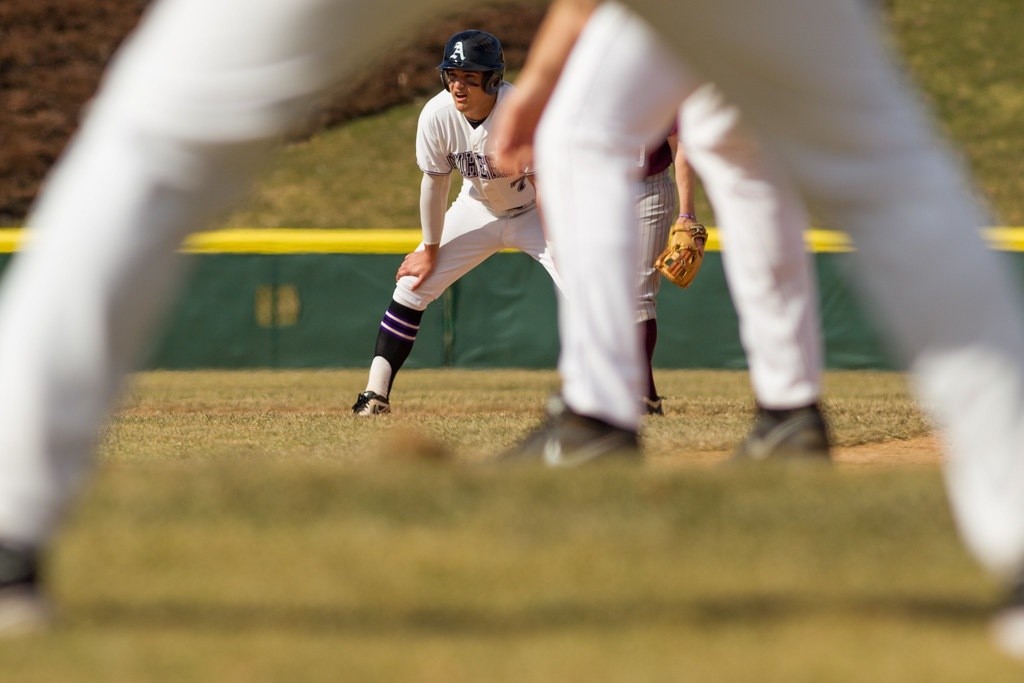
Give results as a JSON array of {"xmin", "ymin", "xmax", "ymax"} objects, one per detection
[{"xmin": 679, "ymin": 213, "xmax": 696, "ymax": 219}]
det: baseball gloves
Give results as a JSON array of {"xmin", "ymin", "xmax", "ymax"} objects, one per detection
[{"xmin": 654, "ymin": 223, "xmax": 709, "ymax": 288}]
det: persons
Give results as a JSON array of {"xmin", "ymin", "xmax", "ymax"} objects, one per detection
[
  {"xmin": 628, "ymin": 113, "xmax": 708, "ymax": 414},
  {"xmin": 487, "ymin": 0, "xmax": 834, "ymax": 466},
  {"xmin": 0, "ymin": 0, "xmax": 1024, "ymax": 655},
  {"xmin": 351, "ymin": 30, "xmax": 557, "ymax": 415}
]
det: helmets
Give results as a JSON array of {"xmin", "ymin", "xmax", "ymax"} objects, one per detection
[{"xmin": 439, "ymin": 30, "xmax": 506, "ymax": 96}]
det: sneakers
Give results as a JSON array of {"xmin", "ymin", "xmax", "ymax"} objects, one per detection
[
  {"xmin": 351, "ymin": 390, "xmax": 392, "ymax": 416},
  {"xmin": 491, "ymin": 395, "xmax": 644, "ymax": 470},
  {"xmin": 721, "ymin": 401, "xmax": 838, "ymax": 478}
]
